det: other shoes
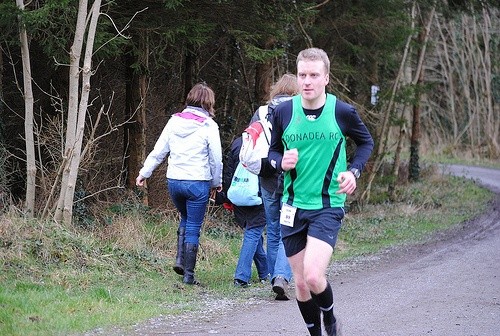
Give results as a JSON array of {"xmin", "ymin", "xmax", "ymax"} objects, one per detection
[
  {"xmin": 273, "ymin": 277, "xmax": 291, "ymax": 300},
  {"xmin": 234, "ymin": 280, "xmax": 251, "ymax": 287}
]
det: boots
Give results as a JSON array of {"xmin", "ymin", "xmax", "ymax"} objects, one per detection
[
  {"xmin": 173, "ymin": 227, "xmax": 184, "ymax": 275},
  {"xmin": 182, "ymin": 243, "xmax": 201, "ymax": 286}
]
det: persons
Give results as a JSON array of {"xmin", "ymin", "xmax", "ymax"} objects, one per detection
[
  {"xmin": 135, "ymin": 83, "xmax": 223, "ymax": 286},
  {"xmin": 267, "ymin": 47, "xmax": 374, "ymax": 336},
  {"xmin": 229, "ymin": 72, "xmax": 299, "ymax": 300}
]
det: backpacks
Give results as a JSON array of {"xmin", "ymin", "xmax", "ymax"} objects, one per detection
[
  {"xmin": 238, "ymin": 105, "xmax": 272, "ymax": 175},
  {"xmin": 227, "ymin": 162, "xmax": 262, "ymax": 207}
]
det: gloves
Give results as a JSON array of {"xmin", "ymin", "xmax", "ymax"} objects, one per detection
[{"xmin": 224, "ymin": 204, "xmax": 232, "ymax": 213}]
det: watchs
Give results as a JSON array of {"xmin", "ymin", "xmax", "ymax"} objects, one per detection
[{"xmin": 347, "ymin": 167, "xmax": 361, "ymax": 179}]
